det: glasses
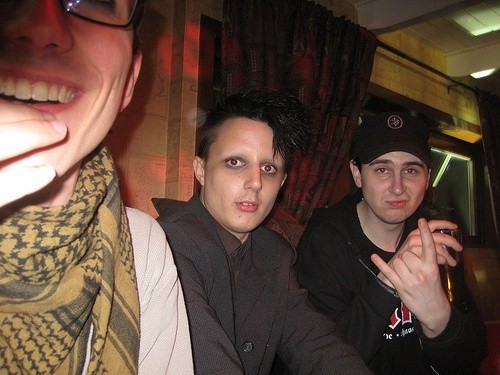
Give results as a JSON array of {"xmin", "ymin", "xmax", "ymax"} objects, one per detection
[{"xmin": 55, "ymin": 0, "xmax": 148, "ymax": 31}]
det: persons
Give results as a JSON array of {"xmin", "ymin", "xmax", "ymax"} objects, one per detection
[
  {"xmin": 294, "ymin": 112, "xmax": 488, "ymax": 374},
  {"xmin": 155, "ymin": 91, "xmax": 372, "ymax": 375},
  {"xmin": 0, "ymin": 1, "xmax": 194, "ymax": 375}
]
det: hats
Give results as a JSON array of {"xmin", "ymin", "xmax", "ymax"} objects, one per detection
[{"xmin": 351, "ymin": 111, "xmax": 433, "ymax": 169}]
